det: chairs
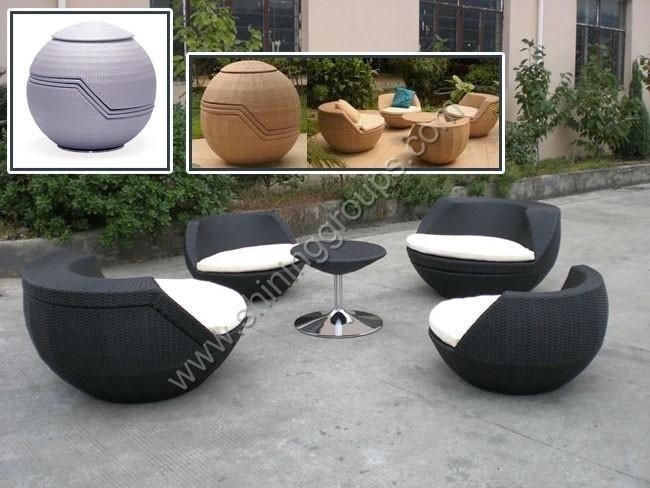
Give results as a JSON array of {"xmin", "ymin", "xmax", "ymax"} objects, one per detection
[
  {"xmin": 406, "ymin": 194, "xmax": 610, "ymax": 393},
  {"xmin": 318, "ymin": 86, "xmax": 500, "ymax": 167},
  {"xmin": 183, "ymin": 211, "xmax": 301, "ymax": 298},
  {"xmin": 19, "ymin": 250, "xmax": 249, "ymax": 404}
]
